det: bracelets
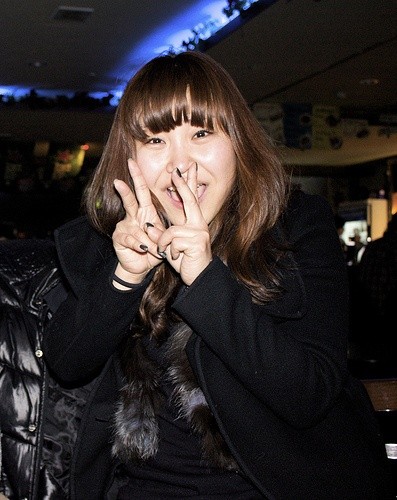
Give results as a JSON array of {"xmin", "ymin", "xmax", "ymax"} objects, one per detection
[{"xmin": 110, "ymin": 270, "xmax": 149, "ymax": 289}]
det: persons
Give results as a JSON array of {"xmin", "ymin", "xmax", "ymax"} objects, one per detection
[{"xmin": 24, "ymin": 51, "xmax": 353, "ymax": 499}]
